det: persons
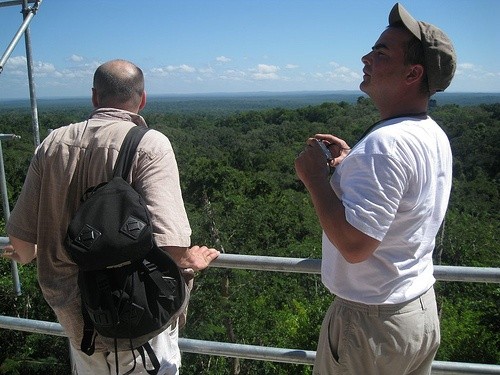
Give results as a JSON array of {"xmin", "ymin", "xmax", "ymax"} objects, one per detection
[
  {"xmin": 1, "ymin": 59, "xmax": 220, "ymax": 375},
  {"xmin": 295, "ymin": 2, "xmax": 457, "ymax": 375}
]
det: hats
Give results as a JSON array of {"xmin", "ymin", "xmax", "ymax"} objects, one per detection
[{"xmin": 388, "ymin": 2, "xmax": 456, "ymax": 93}]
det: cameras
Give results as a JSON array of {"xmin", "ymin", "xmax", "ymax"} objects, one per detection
[{"xmin": 317, "ymin": 141, "xmax": 333, "ymax": 161}]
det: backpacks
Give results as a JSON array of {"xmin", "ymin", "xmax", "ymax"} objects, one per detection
[{"xmin": 63, "ymin": 125, "xmax": 189, "ymax": 356}]
